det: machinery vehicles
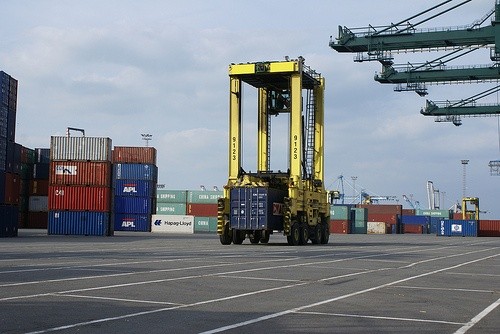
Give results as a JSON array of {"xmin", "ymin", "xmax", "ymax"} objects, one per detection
[{"xmin": 217, "ymin": 55, "xmax": 330, "ymax": 245}]
